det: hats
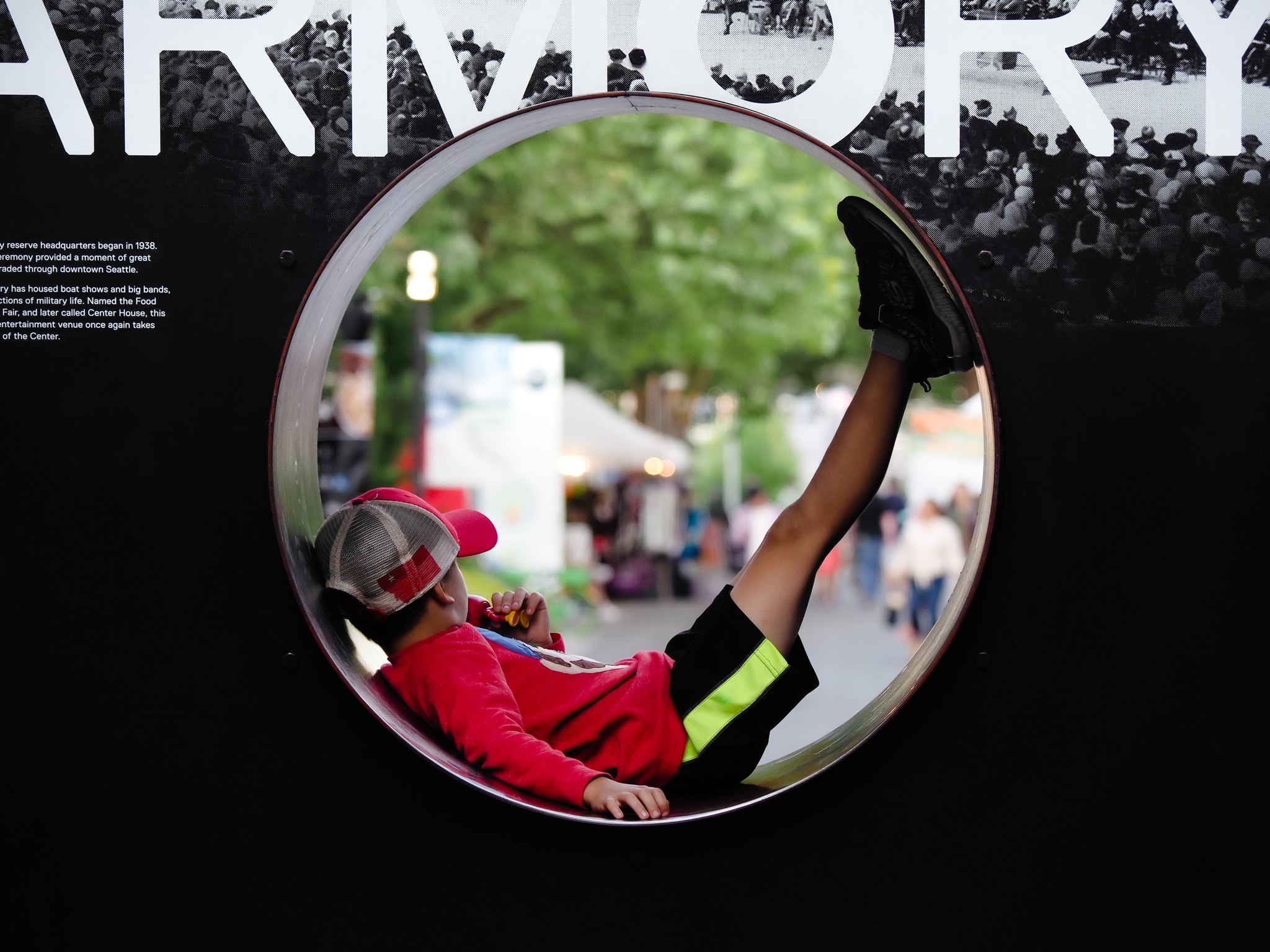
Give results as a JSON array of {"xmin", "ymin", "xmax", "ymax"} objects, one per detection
[{"xmin": 312, "ymin": 485, "xmax": 498, "ymax": 619}]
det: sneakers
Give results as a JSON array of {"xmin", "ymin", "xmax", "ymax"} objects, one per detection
[{"xmin": 837, "ymin": 191, "xmax": 976, "ymax": 393}]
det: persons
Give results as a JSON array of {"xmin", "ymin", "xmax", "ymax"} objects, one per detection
[
  {"xmin": 311, "ymin": 193, "xmax": 975, "ymax": 817},
  {"xmin": 2, "ymin": 0, "xmax": 1268, "ymax": 337},
  {"xmin": 564, "ymin": 463, "xmax": 979, "ymax": 650}
]
